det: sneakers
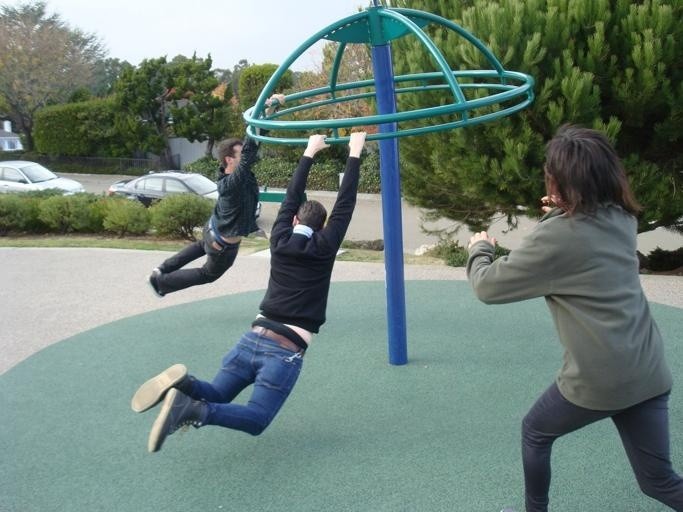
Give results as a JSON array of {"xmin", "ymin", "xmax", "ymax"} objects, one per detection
[{"xmin": 149, "ymin": 267, "xmax": 164, "ymax": 298}]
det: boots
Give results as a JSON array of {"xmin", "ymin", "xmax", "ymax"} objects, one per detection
[
  {"xmin": 148, "ymin": 387, "xmax": 209, "ymax": 451},
  {"xmin": 131, "ymin": 364, "xmax": 196, "ymax": 414}
]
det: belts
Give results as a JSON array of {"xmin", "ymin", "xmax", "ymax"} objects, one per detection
[
  {"xmin": 252, "ymin": 326, "xmax": 300, "ymax": 353},
  {"xmin": 208, "ymin": 215, "xmax": 224, "ymax": 251}
]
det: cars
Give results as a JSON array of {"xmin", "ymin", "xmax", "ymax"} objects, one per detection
[
  {"xmin": 0, "ymin": 160, "xmax": 87, "ymax": 194},
  {"xmin": 108, "ymin": 173, "xmax": 261, "ymax": 218}
]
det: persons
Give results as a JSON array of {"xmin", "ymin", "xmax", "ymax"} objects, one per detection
[
  {"xmin": 466, "ymin": 122, "xmax": 683, "ymax": 512},
  {"xmin": 131, "ymin": 130, "xmax": 368, "ymax": 452},
  {"xmin": 149, "ymin": 93, "xmax": 286, "ymax": 296}
]
